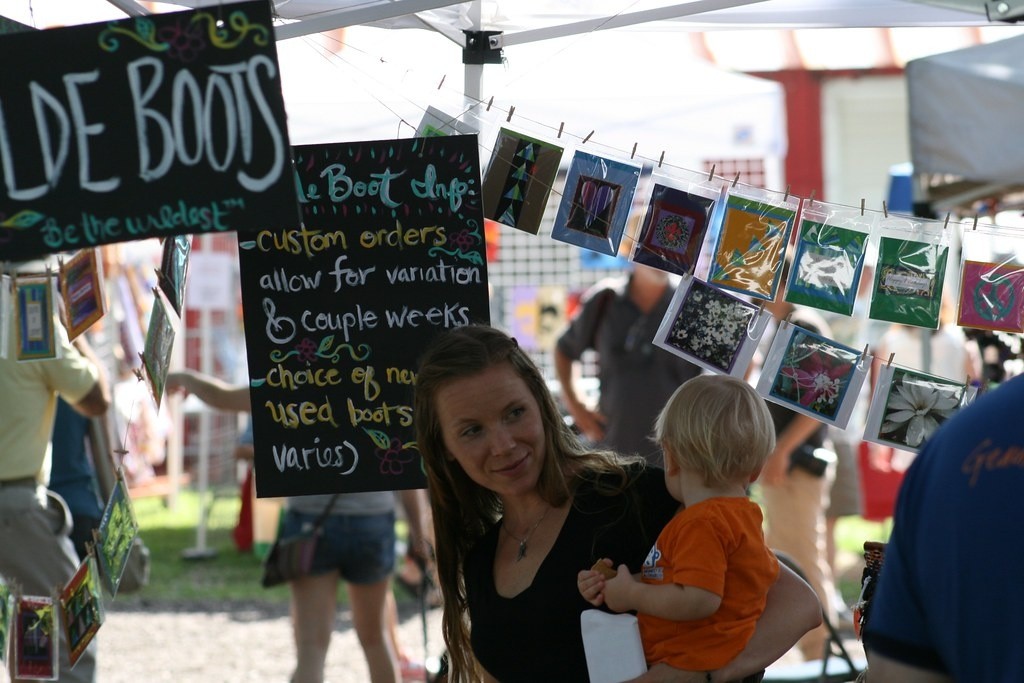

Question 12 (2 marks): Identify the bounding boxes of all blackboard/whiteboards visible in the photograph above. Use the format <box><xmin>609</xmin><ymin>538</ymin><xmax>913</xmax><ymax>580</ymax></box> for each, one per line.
<box><xmin>0</xmin><ymin>0</ymin><xmax>291</xmax><ymax>270</ymax></box>
<box><xmin>237</xmin><ymin>132</ymin><xmax>488</xmax><ymax>504</ymax></box>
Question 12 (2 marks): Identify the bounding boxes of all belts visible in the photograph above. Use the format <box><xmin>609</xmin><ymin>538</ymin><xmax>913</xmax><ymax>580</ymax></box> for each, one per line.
<box><xmin>0</xmin><ymin>477</ymin><xmax>39</xmax><ymax>487</ymax></box>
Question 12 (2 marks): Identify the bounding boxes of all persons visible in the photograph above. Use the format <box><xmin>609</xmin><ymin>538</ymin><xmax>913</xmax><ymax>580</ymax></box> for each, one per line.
<box><xmin>233</xmin><ymin>417</ymin><xmax>255</xmax><ymax>460</ymax></box>
<box><xmin>551</xmin><ymin>254</ymin><xmax>704</xmax><ymax>461</ymax></box>
<box><xmin>578</xmin><ymin>374</ymin><xmax>778</xmax><ymax>668</ymax></box>
<box><xmin>861</xmin><ymin>372</ymin><xmax>1024</xmax><ymax>683</ymax></box>
<box><xmin>753</xmin><ymin>245</ymin><xmax>829</xmax><ymax>659</ymax></box>
<box><xmin>0</xmin><ymin>257</ymin><xmax>111</xmax><ymax>683</ymax></box>
<box><xmin>166</xmin><ymin>370</ymin><xmax>432</xmax><ymax>683</ymax></box>
<box><xmin>417</xmin><ymin>326</ymin><xmax>824</xmax><ymax>683</ymax></box>
<box><xmin>828</xmin><ymin>260</ymin><xmax>1024</xmax><ymax>576</ymax></box>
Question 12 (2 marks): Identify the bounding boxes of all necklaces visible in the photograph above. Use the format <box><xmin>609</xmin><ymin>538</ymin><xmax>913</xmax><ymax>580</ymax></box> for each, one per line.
<box><xmin>502</xmin><ymin>506</ymin><xmax>550</xmax><ymax>560</ymax></box>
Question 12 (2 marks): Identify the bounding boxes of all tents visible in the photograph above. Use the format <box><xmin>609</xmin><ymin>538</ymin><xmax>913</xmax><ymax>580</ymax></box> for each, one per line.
<box><xmin>905</xmin><ymin>33</ymin><xmax>1023</xmax><ymax>377</ymax></box>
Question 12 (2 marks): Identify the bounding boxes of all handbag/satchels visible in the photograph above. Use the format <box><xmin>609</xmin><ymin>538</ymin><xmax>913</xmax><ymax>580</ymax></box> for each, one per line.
<box><xmin>263</xmin><ymin>530</ymin><xmax>313</xmax><ymax>587</ymax></box>
